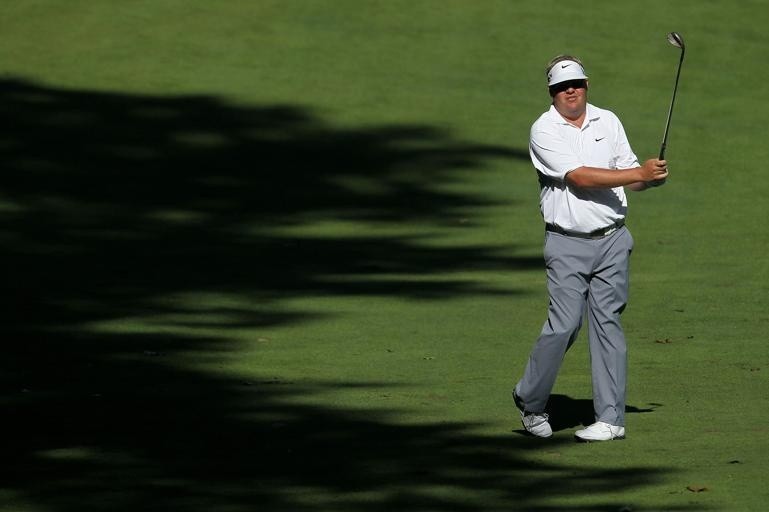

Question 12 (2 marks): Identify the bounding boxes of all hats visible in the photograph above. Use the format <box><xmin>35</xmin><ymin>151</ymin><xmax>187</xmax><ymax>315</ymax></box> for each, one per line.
<box><xmin>547</xmin><ymin>60</ymin><xmax>589</xmax><ymax>86</ymax></box>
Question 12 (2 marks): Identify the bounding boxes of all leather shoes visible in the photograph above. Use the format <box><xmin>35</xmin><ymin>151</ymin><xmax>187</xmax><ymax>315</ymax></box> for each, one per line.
<box><xmin>511</xmin><ymin>386</ymin><xmax>552</xmax><ymax>438</ymax></box>
<box><xmin>574</xmin><ymin>423</ymin><xmax>626</xmax><ymax>441</ymax></box>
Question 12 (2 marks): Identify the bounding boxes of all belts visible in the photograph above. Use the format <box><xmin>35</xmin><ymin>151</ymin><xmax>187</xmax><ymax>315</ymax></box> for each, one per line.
<box><xmin>545</xmin><ymin>221</ymin><xmax>625</xmax><ymax>240</ymax></box>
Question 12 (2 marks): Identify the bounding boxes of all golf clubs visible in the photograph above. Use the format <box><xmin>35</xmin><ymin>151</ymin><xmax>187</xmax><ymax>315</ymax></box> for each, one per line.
<box><xmin>658</xmin><ymin>32</ymin><xmax>686</xmax><ymax>162</ymax></box>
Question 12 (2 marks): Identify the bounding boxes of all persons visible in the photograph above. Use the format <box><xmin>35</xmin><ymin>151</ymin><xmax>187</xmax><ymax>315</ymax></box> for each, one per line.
<box><xmin>511</xmin><ymin>55</ymin><xmax>667</xmax><ymax>442</ymax></box>
